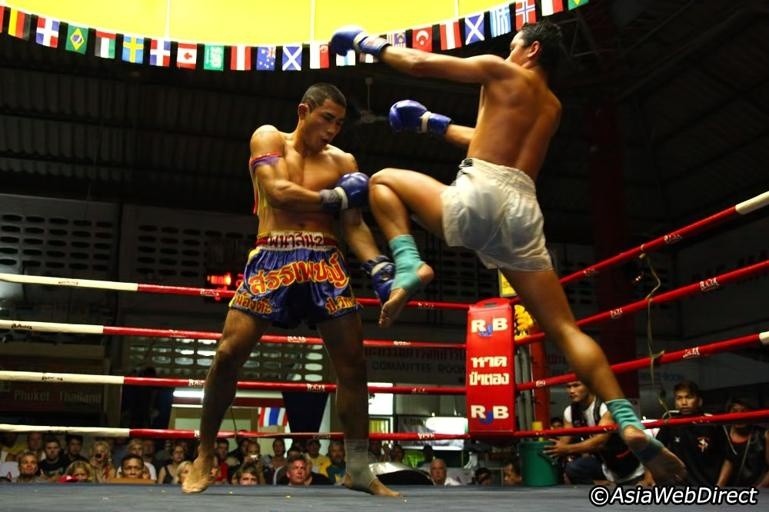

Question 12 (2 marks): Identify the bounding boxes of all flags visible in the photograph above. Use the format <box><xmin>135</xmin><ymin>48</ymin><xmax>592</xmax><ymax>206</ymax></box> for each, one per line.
<box><xmin>0</xmin><ymin>0</ymin><xmax>589</xmax><ymax>72</ymax></box>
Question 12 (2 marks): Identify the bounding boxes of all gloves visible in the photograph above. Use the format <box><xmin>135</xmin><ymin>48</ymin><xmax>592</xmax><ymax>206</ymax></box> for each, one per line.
<box><xmin>329</xmin><ymin>23</ymin><xmax>391</xmax><ymax>65</ymax></box>
<box><xmin>358</xmin><ymin>254</ymin><xmax>398</xmax><ymax>309</ymax></box>
<box><xmin>386</xmin><ymin>98</ymin><xmax>454</xmax><ymax>142</ymax></box>
<box><xmin>322</xmin><ymin>169</ymin><xmax>370</xmax><ymax>214</ymax></box>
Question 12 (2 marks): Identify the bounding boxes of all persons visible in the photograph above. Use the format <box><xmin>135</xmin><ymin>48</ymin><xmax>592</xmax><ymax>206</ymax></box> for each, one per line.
<box><xmin>0</xmin><ymin>365</ymin><xmax>765</xmax><ymax>488</ymax></box>
<box><xmin>329</xmin><ymin>22</ymin><xmax>689</xmax><ymax>487</ymax></box>
<box><xmin>182</xmin><ymin>83</ymin><xmax>402</xmax><ymax>497</ymax></box>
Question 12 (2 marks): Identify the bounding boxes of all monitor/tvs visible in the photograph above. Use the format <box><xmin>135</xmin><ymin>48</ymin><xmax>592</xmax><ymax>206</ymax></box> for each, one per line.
<box><xmin>367</xmin><ymin>380</ymin><xmax>395</xmax><ymax>416</ymax></box>
<box><xmin>424</xmin><ymin>416</ymin><xmax>469</xmax><ymax>452</ymax></box>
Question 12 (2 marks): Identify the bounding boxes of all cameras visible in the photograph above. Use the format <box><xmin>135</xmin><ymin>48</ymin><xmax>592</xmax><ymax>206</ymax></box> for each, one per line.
<box><xmin>96</xmin><ymin>453</ymin><xmax>101</xmax><ymax>458</ymax></box>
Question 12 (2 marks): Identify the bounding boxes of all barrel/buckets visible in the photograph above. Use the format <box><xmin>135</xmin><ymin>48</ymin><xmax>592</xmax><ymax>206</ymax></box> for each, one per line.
<box><xmin>520</xmin><ymin>440</ymin><xmax>561</xmax><ymax>487</ymax></box>
